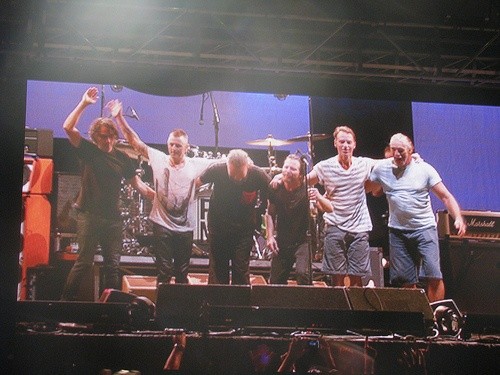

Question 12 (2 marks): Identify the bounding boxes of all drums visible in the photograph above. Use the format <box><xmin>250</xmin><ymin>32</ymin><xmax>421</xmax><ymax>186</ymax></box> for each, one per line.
<box><xmin>125</xmin><ymin>182</ymin><xmax>153</xmax><ymax>235</ymax></box>
<box><xmin>257</xmin><ymin>167</ymin><xmax>282</xmax><ymax>205</ymax></box>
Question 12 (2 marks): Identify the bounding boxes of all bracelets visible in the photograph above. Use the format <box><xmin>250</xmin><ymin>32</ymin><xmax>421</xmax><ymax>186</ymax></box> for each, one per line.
<box><xmin>174</xmin><ymin>343</ymin><xmax>185</xmax><ymax>350</ymax></box>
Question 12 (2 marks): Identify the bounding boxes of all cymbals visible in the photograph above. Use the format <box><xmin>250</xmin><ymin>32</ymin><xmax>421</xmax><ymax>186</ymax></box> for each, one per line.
<box><xmin>284</xmin><ymin>130</ymin><xmax>331</xmax><ymax>145</ymax></box>
<box><xmin>114</xmin><ymin>141</ymin><xmax>148</xmax><ymax>163</ymax></box>
<box><xmin>246</xmin><ymin>134</ymin><xmax>290</xmax><ymax>149</ymax></box>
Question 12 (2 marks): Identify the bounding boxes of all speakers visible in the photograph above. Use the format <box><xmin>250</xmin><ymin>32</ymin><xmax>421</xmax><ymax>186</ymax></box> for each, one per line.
<box><xmin>348</xmin><ymin>286</ymin><xmax>434</xmax><ymax>332</ymax></box>
<box><xmin>436</xmin><ymin>237</ymin><xmax>500</xmax><ymax>324</ymax></box>
<box><xmin>249</xmin><ymin>284</ymin><xmax>351</xmax><ymax>324</ymax></box>
<box><xmin>153</xmin><ymin>283</ymin><xmax>250</xmax><ymax>328</ymax></box>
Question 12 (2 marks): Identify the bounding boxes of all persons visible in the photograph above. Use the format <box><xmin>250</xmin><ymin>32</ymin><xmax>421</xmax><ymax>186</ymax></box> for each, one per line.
<box><xmin>164</xmin><ymin>335</ymin><xmax>186</xmax><ymax>375</ymax></box>
<box><xmin>106</xmin><ymin>99</ymin><xmax>228</xmax><ymax>283</ymax></box>
<box><xmin>270</xmin><ymin>126</ymin><xmax>422</xmax><ymax>288</ymax></box>
<box><xmin>264</xmin><ymin>154</ymin><xmax>334</xmax><ymax>285</ymax></box>
<box><xmin>61</xmin><ymin>87</ymin><xmax>156</xmax><ymax>301</ymax></box>
<box><xmin>276</xmin><ymin>336</ymin><xmax>500</xmax><ymax>375</ymax></box>
<box><xmin>366</xmin><ymin>132</ymin><xmax>466</xmax><ymax>303</ymax></box>
<box><xmin>196</xmin><ymin>149</ymin><xmax>273</xmax><ymax>285</ymax></box>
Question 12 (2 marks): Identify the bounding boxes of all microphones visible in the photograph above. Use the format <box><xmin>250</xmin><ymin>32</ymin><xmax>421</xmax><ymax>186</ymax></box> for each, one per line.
<box><xmin>129</xmin><ymin>106</ymin><xmax>139</xmax><ymax>120</ymax></box>
<box><xmin>301</xmin><ymin>156</ymin><xmax>310</xmax><ymax>165</ymax></box>
<box><xmin>200</xmin><ymin>103</ymin><xmax>204</xmax><ymax>125</ymax></box>
